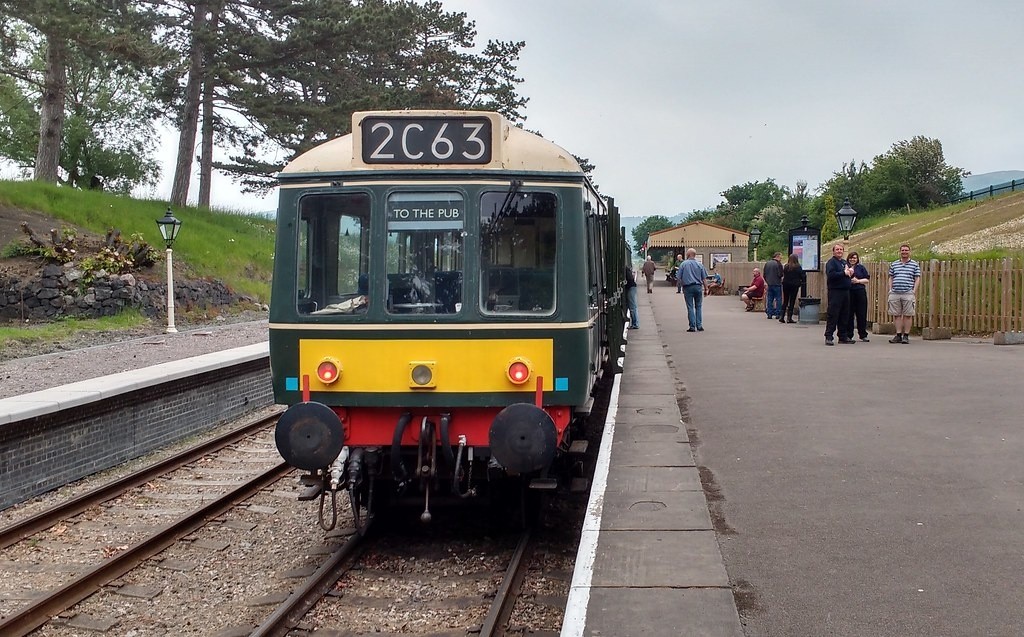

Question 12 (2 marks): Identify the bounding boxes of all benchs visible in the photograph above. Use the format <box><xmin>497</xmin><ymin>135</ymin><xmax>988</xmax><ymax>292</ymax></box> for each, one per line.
<box><xmin>354</xmin><ymin>264</ymin><xmax>557</xmax><ymax>313</ymax></box>
<box><xmin>714</xmin><ymin>278</ymin><xmax>726</xmax><ymax>294</ymax></box>
<box><xmin>751</xmin><ymin>285</ymin><xmax>766</xmax><ymax>311</ymax></box>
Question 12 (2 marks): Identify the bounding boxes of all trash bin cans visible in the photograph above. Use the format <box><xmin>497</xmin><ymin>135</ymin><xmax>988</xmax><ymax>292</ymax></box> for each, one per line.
<box><xmin>739</xmin><ymin>286</ymin><xmax>750</xmax><ymax>301</ymax></box>
<box><xmin>798</xmin><ymin>298</ymin><xmax>821</xmax><ymax>324</ymax></box>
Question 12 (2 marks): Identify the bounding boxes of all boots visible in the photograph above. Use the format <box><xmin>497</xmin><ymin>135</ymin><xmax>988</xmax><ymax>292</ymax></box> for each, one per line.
<box><xmin>786</xmin><ymin>311</ymin><xmax>796</xmax><ymax>323</ymax></box>
<box><xmin>779</xmin><ymin>310</ymin><xmax>785</xmax><ymax>323</ymax></box>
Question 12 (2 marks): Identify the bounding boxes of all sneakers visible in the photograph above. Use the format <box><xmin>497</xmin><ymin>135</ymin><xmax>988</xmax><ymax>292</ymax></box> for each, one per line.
<box><xmin>889</xmin><ymin>337</ymin><xmax>902</xmax><ymax>343</ymax></box>
<box><xmin>902</xmin><ymin>337</ymin><xmax>909</xmax><ymax>344</ymax></box>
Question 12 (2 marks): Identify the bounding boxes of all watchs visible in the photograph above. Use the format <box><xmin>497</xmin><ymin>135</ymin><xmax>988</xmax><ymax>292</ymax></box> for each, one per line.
<box><xmin>704</xmin><ymin>288</ymin><xmax>708</xmax><ymax>290</ymax></box>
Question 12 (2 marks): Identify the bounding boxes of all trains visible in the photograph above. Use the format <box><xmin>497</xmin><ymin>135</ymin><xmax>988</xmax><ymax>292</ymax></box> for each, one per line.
<box><xmin>268</xmin><ymin>107</ymin><xmax>633</xmax><ymax>539</ymax></box>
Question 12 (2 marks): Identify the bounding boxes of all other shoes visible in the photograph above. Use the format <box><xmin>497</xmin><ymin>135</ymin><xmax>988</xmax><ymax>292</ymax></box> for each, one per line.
<box><xmin>863</xmin><ymin>337</ymin><xmax>869</xmax><ymax>342</ymax></box>
<box><xmin>687</xmin><ymin>328</ymin><xmax>695</xmax><ymax>332</ymax></box>
<box><xmin>838</xmin><ymin>337</ymin><xmax>855</xmax><ymax>344</ymax></box>
<box><xmin>768</xmin><ymin>314</ymin><xmax>772</xmax><ymax>319</ymax></box>
<box><xmin>746</xmin><ymin>304</ymin><xmax>754</xmax><ymax>311</ymax></box>
<box><xmin>627</xmin><ymin>326</ymin><xmax>639</xmax><ymax>329</ymax></box>
<box><xmin>825</xmin><ymin>337</ymin><xmax>834</xmax><ymax>345</ymax></box>
<box><xmin>776</xmin><ymin>315</ymin><xmax>779</xmax><ymax>319</ymax></box>
<box><xmin>697</xmin><ymin>326</ymin><xmax>704</xmax><ymax>331</ymax></box>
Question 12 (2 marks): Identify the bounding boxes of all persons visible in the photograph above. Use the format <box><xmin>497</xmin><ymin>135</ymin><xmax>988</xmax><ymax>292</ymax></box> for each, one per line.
<box><xmin>674</xmin><ymin>254</ymin><xmax>684</xmax><ymax>294</ymax></box>
<box><xmin>741</xmin><ymin>267</ymin><xmax>764</xmax><ymax>312</ymax></box>
<box><xmin>708</xmin><ymin>270</ymin><xmax>723</xmax><ymax>295</ymax></box>
<box><xmin>824</xmin><ymin>242</ymin><xmax>856</xmax><ymax>346</ymax></box>
<box><xmin>624</xmin><ymin>266</ymin><xmax>640</xmax><ymax>330</ymax></box>
<box><xmin>779</xmin><ymin>253</ymin><xmax>806</xmax><ymax>323</ymax></box>
<box><xmin>642</xmin><ymin>255</ymin><xmax>657</xmax><ymax>294</ymax></box>
<box><xmin>887</xmin><ymin>245</ymin><xmax>921</xmax><ymax>344</ymax></box>
<box><xmin>847</xmin><ymin>252</ymin><xmax>871</xmax><ymax>343</ymax></box>
<box><xmin>762</xmin><ymin>252</ymin><xmax>784</xmax><ymax>320</ymax></box>
<box><xmin>676</xmin><ymin>248</ymin><xmax>709</xmax><ymax>333</ymax></box>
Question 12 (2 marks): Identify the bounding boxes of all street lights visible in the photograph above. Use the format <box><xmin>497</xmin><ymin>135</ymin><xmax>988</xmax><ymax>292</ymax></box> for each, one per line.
<box><xmin>157</xmin><ymin>206</ymin><xmax>183</xmax><ymax>334</ymax></box>
<box><xmin>836</xmin><ymin>199</ymin><xmax>858</xmax><ymax>261</ymax></box>
<box><xmin>749</xmin><ymin>224</ymin><xmax>762</xmax><ymax>262</ymax></box>
